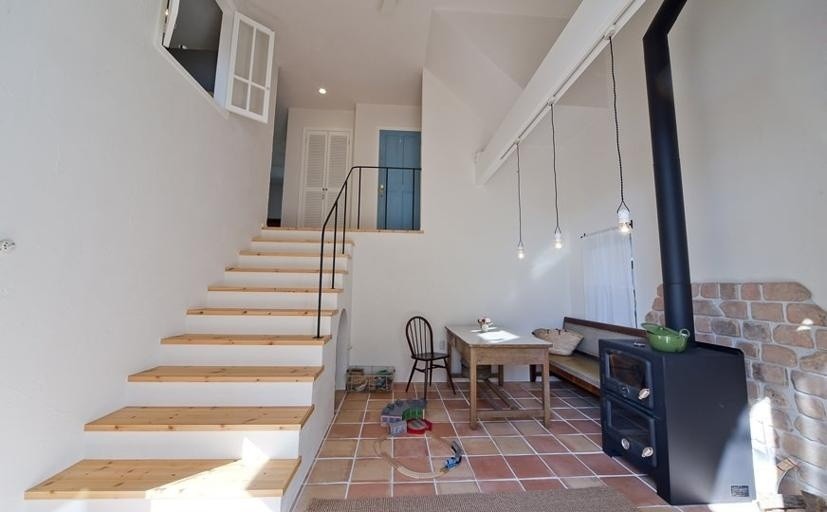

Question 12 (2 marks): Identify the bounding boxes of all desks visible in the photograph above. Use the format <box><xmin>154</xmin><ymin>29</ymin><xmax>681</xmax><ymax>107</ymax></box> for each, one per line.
<box><xmin>445</xmin><ymin>324</ymin><xmax>553</xmax><ymax>431</ymax></box>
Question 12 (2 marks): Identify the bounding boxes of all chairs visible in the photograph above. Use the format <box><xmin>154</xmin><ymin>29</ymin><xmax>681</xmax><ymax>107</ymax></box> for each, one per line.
<box><xmin>406</xmin><ymin>316</ymin><xmax>456</xmax><ymax>401</ymax></box>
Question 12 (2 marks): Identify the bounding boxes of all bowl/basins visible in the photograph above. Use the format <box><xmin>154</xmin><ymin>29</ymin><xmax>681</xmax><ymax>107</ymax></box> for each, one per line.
<box><xmin>648</xmin><ymin>332</ymin><xmax>691</xmax><ymax>352</ymax></box>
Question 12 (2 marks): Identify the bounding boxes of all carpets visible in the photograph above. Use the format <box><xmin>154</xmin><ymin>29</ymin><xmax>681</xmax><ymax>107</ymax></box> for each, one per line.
<box><xmin>305</xmin><ymin>486</ymin><xmax>637</xmax><ymax>511</ymax></box>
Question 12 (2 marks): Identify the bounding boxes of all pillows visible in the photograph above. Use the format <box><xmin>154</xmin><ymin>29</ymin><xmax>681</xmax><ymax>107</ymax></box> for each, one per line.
<box><xmin>532</xmin><ymin>329</ymin><xmax>585</xmax><ymax>357</ymax></box>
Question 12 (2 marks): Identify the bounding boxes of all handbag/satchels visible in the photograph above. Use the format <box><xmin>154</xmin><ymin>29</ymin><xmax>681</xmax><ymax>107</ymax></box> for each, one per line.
<box><xmin>532</xmin><ymin>328</ymin><xmax>584</xmax><ymax>355</ymax></box>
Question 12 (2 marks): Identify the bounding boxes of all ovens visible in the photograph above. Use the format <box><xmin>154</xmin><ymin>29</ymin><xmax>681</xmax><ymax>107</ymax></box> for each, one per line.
<box><xmin>599</xmin><ymin>340</ymin><xmax>753</xmax><ymax>505</ymax></box>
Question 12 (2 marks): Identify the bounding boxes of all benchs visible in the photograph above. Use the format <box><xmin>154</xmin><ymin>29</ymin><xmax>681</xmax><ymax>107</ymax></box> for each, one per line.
<box><xmin>529</xmin><ymin>317</ymin><xmax>647</xmax><ymax>398</ymax></box>
